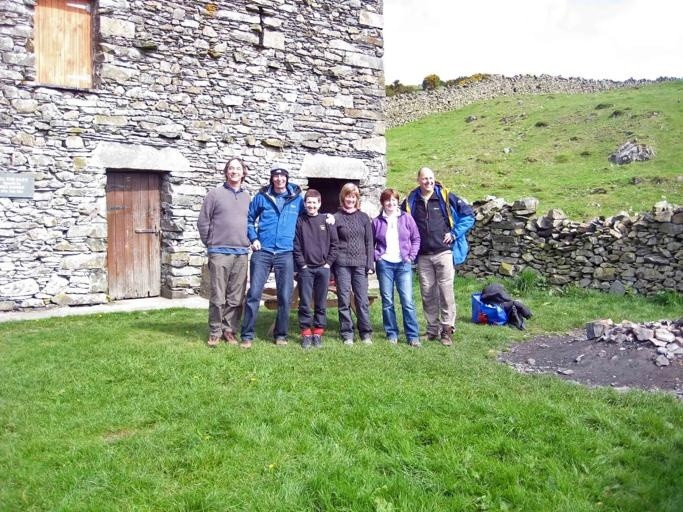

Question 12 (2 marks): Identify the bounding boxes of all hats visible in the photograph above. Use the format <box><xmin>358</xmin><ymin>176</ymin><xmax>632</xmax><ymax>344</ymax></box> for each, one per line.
<box><xmin>271</xmin><ymin>164</ymin><xmax>289</xmax><ymax>176</ymax></box>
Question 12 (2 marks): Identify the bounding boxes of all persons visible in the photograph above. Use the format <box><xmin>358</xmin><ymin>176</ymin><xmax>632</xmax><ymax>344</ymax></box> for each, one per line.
<box><xmin>399</xmin><ymin>167</ymin><xmax>476</xmax><ymax>346</ymax></box>
<box><xmin>197</xmin><ymin>158</ymin><xmax>251</xmax><ymax>347</ymax></box>
<box><xmin>294</xmin><ymin>189</ymin><xmax>340</xmax><ymax>348</ymax></box>
<box><xmin>331</xmin><ymin>183</ymin><xmax>376</xmax><ymax>345</ymax></box>
<box><xmin>371</xmin><ymin>189</ymin><xmax>422</xmax><ymax>349</ymax></box>
<box><xmin>239</xmin><ymin>163</ymin><xmax>335</xmax><ymax>348</ymax></box>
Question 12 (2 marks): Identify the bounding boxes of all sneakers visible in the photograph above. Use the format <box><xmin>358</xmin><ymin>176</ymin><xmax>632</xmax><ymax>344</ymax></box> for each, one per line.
<box><xmin>208</xmin><ymin>335</ymin><xmax>219</xmax><ymax>347</ymax></box>
<box><xmin>362</xmin><ymin>338</ymin><xmax>372</xmax><ymax>343</ymax></box>
<box><xmin>240</xmin><ymin>337</ymin><xmax>252</xmax><ymax>348</ymax></box>
<box><xmin>387</xmin><ymin>339</ymin><xmax>397</xmax><ymax>344</ymax></box>
<box><xmin>302</xmin><ymin>335</ymin><xmax>313</xmax><ymax>348</ymax></box>
<box><xmin>419</xmin><ymin>334</ymin><xmax>434</xmax><ymax>341</ymax></box>
<box><xmin>410</xmin><ymin>342</ymin><xmax>422</xmax><ymax>348</ymax></box>
<box><xmin>440</xmin><ymin>335</ymin><xmax>453</xmax><ymax>346</ymax></box>
<box><xmin>225</xmin><ymin>333</ymin><xmax>238</xmax><ymax>345</ymax></box>
<box><xmin>313</xmin><ymin>334</ymin><xmax>323</xmax><ymax>349</ymax></box>
<box><xmin>276</xmin><ymin>336</ymin><xmax>287</xmax><ymax>345</ymax></box>
<box><xmin>343</xmin><ymin>338</ymin><xmax>354</xmax><ymax>345</ymax></box>
